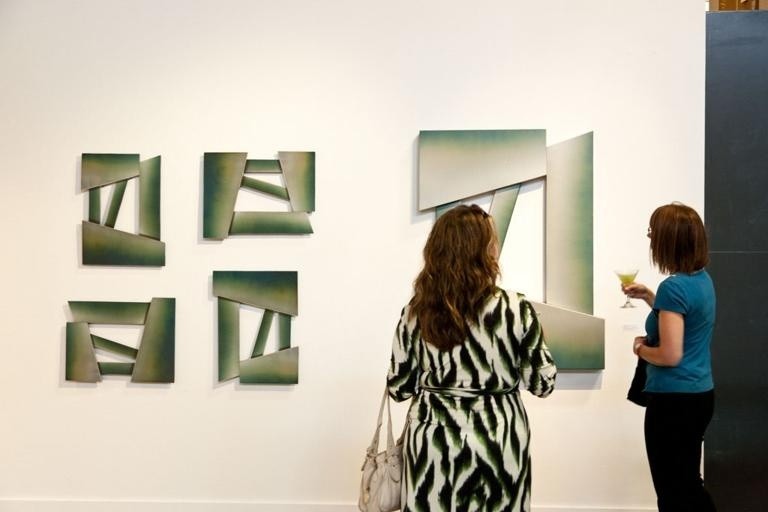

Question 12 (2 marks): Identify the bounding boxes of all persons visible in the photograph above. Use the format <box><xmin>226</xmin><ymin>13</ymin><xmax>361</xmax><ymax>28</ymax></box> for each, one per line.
<box><xmin>384</xmin><ymin>202</ymin><xmax>559</xmax><ymax>511</ymax></box>
<box><xmin>619</xmin><ymin>202</ymin><xmax>719</xmax><ymax>511</ymax></box>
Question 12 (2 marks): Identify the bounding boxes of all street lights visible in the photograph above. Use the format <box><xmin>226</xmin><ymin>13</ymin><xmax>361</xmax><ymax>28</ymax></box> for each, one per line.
<box><xmin>354</xmin><ymin>385</ymin><xmax>402</xmax><ymax>512</ymax></box>
<box><xmin>626</xmin><ymin>356</ymin><xmax>648</xmax><ymax>407</ymax></box>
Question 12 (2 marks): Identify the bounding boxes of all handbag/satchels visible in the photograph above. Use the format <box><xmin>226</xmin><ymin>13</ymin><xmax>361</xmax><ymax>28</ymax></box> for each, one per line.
<box><xmin>635</xmin><ymin>342</ymin><xmax>642</xmax><ymax>355</ymax></box>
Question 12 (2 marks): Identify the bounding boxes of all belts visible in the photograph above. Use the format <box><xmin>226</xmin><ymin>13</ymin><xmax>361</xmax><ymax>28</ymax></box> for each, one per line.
<box><xmin>615</xmin><ymin>268</ymin><xmax>639</xmax><ymax>309</ymax></box>
